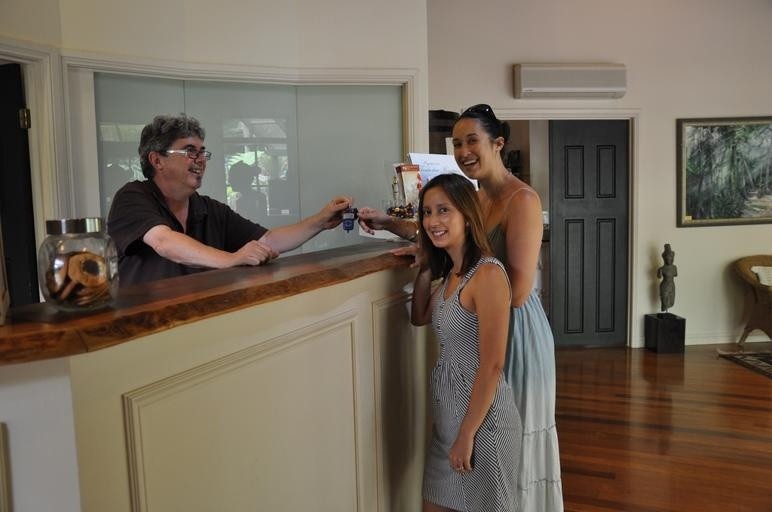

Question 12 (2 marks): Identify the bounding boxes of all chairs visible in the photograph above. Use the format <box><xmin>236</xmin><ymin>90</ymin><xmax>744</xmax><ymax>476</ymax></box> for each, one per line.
<box><xmin>733</xmin><ymin>255</ymin><xmax>772</xmax><ymax>352</ymax></box>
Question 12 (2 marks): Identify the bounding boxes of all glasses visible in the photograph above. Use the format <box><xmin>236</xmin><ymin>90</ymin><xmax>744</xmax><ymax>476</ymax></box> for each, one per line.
<box><xmin>165</xmin><ymin>147</ymin><xmax>212</xmax><ymax>159</ymax></box>
<box><xmin>468</xmin><ymin>105</ymin><xmax>496</xmax><ymax>119</ymax></box>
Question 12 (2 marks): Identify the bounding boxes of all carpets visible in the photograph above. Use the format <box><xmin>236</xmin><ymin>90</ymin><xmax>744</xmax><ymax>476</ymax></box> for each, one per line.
<box><xmin>718</xmin><ymin>350</ymin><xmax>771</xmax><ymax>380</ymax></box>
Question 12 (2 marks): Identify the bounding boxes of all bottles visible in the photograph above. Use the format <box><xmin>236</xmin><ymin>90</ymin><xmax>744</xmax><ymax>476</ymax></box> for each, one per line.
<box><xmin>392</xmin><ymin>176</ymin><xmax>399</xmax><ymax>201</ymax></box>
<box><xmin>38</xmin><ymin>217</ymin><xmax>120</xmax><ymax>314</ymax></box>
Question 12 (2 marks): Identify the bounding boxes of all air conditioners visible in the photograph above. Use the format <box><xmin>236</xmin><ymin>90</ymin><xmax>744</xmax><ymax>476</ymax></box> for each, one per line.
<box><xmin>512</xmin><ymin>62</ymin><xmax>627</xmax><ymax>99</ymax></box>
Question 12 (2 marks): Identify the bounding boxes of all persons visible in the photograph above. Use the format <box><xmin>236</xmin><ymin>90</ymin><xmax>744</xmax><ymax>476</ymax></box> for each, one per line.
<box><xmin>656</xmin><ymin>242</ymin><xmax>678</xmax><ymax>311</ymax></box>
<box><xmin>388</xmin><ymin>173</ymin><xmax>523</xmax><ymax>511</ymax></box>
<box><xmin>228</xmin><ymin>160</ymin><xmax>267</xmax><ymax>218</ymax></box>
<box><xmin>108</xmin><ymin>112</ymin><xmax>354</xmax><ymax>289</ymax></box>
<box><xmin>357</xmin><ymin>105</ymin><xmax>563</xmax><ymax>512</ymax></box>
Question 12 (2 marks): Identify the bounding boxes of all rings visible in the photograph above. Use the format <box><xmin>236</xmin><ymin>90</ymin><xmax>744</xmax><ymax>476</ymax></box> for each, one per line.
<box><xmin>460</xmin><ymin>467</ymin><xmax>464</xmax><ymax>469</ymax></box>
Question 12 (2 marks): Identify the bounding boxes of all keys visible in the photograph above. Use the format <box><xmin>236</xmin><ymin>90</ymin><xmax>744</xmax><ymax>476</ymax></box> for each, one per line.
<box><xmin>342</xmin><ymin>206</ymin><xmax>359</xmax><ymax>233</ymax></box>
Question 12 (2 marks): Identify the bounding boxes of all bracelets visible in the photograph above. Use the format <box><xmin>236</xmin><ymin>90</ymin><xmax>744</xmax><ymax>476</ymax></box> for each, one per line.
<box><xmin>409</xmin><ymin>220</ymin><xmax>419</xmax><ymax>242</ymax></box>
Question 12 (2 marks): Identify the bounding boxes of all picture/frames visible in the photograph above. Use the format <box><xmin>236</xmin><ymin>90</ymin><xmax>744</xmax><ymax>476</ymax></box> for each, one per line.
<box><xmin>676</xmin><ymin>117</ymin><xmax>771</xmax><ymax>228</ymax></box>
